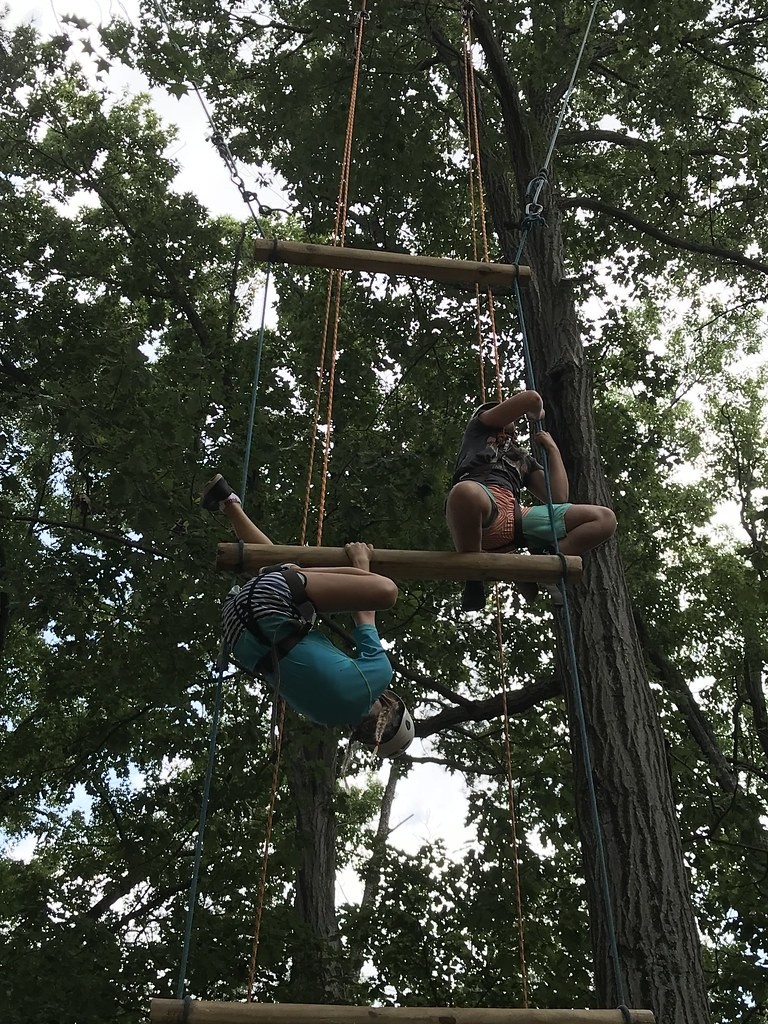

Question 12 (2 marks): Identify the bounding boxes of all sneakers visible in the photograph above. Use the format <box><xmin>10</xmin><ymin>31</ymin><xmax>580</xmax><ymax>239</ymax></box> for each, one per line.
<box><xmin>200</xmin><ymin>474</ymin><xmax>236</xmax><ymax>511</ymax></box>
<box><xmin>260</xmin><ymin>561</ymin><xmax>296</xmax><ymax>574</ymax></box>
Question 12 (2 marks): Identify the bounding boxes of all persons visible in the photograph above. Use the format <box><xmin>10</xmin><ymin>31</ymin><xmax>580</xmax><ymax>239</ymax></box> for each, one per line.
<box><xmin>446</xmin><ymin>391</ymin><xmax>617</xmax><ymax>611</ymax></box>
<box><xmin>200</xmin><ymin>474</ymin><xmax>414</xmax><ymax>759</ymax></box>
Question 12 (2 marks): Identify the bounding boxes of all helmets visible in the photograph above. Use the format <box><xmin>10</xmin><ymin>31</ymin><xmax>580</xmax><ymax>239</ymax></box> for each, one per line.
<box><xmin>356</xmin><ymin>690</ymin><xmax>415</xmax><ymax>758</ymax></box>
<box><xmin>470</xmin><ymin>401</ymin><xmax>500</xmax><ymax>418</ymax></box>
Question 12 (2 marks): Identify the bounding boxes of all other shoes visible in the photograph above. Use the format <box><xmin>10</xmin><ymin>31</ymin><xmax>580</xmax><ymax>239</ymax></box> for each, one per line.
<box><xmin>516</xmin><ymin>581</ymin><xmax>539</xmax><ymax>601</ymax></box>
<box><xmin>461</xmin><ymin>581</ymin><xmax>486</xmax><ymax>610</ymax></box>
